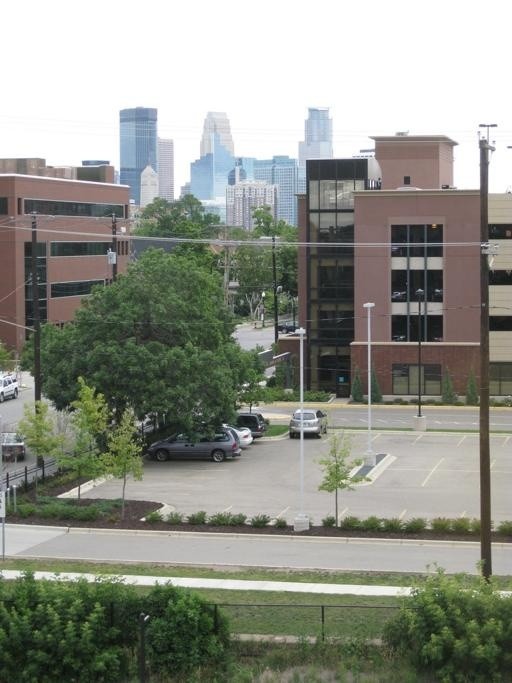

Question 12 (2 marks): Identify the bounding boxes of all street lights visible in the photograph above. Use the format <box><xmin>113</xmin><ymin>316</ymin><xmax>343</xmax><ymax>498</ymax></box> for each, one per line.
<box><xmin>294</xmin><ymin>327</ymin><xmax>310</xmax><ymax>531</ymax></box>
<box><xmin>278</xmin><ymin>323</ymin><xmax>297</xmax><ymax>333</ymax></box>
<box><xmin>415</xmin><ymin>288</ymin><xmax>425</xmax><ymax>417</ymax></box>
<box><xmin>274</xmin><ymin>285</ymin><xmax>283</xmax><ymax>341</ymax></box>
<box><xmin>363</xmin><ymin>301</ymin><xmax>376</xmax><ymax>466</ymax></box>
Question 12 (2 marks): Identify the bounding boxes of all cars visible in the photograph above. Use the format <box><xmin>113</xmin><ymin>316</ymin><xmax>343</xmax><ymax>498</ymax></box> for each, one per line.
<box><xmin>0</xmin><ymin>431</ymin><xmax>25</xmax><ymax>462</ymax></box>
<box><xmin>289</xmin><ymin>408</ymin><xmax>328</xmax><ymax>439</ymax></box>
<box><xmin>0</xmin><ymin>374</ymin><xmax>19</xmax><ymax>402</ymax></box>
<box><xmin>146</xmin><ymin>413</ymin><xmax>266</xmax><ymax>462</ymax></box>
